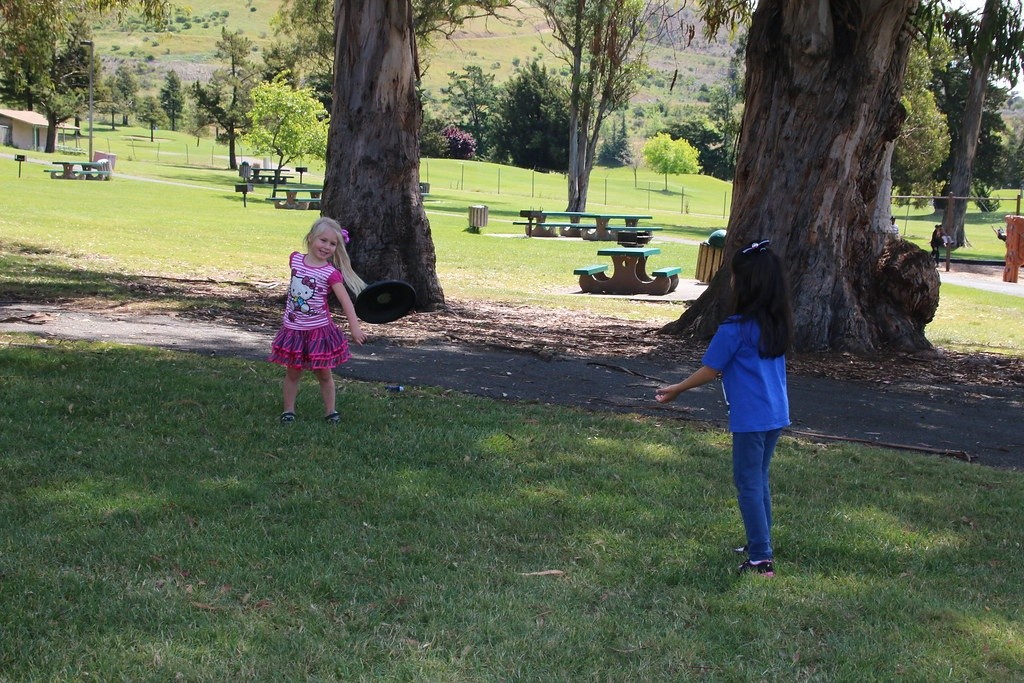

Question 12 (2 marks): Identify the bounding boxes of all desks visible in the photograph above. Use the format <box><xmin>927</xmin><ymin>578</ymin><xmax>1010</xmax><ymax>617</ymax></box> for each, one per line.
<box><xmin>578</xmin><ymin>247</ymin><xmax>678</xmax><ymax>296</ymax></box>
<box><xmin>525</xmin><ymin>211</ymin><xmax>652</xmax><ymax>241</ymax></box>
<box><xmin>274</xmin><ymin>188</ymin><xmax>323</xmax><ymax>210</ymax></box>
<box><xmin>250</xmin><ymin>167</ymin><xmax>290</xmax><ymax>183</ymax></box>
<box><xmin>51</xmin><ymin>161</ymin><xmax>109</xmax><ymax>181</ymax></box>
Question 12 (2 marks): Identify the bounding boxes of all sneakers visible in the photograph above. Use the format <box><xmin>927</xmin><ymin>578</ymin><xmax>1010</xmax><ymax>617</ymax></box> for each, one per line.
<box><xmin>734</xmin><ymin>545</ymin><xmax>748</xmax><ymax>554</ymax></box>
<box><xmin>738</xmin><ymin>558</ymin><xmax>773</xmax><ymax>577</ymax></box>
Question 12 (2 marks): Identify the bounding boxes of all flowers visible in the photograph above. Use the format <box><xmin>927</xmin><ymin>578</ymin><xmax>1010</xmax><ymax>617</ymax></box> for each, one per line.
<box><xmin>340</xmin><ymin>228</ymin><xmax>351</xmax><ymax>243</ymax></box>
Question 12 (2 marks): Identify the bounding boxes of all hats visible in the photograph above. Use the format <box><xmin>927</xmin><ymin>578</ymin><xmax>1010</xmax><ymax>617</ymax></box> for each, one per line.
<box><xmin>935</xmin><ymin>225</ymin><xmax>941</xmax><ymax>229</ymax></box>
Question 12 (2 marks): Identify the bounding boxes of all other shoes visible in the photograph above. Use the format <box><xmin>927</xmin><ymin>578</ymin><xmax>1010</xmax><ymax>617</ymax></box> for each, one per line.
<box><xmin>280</xmin><ymin>412</ymin><xmax>296</xmax><ymax>422</ymax></box>
<box><xmin>325</xmin><ymin>413</ymin><xmax>341</xmax><ymax>424</ymax></box>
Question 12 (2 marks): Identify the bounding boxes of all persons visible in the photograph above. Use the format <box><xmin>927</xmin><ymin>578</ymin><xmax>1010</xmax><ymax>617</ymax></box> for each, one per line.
<box><xmin>931</xmin><ymin>225</ymin><xmax>941</xmax><ymax>268</ymax></box>
<box><xmin>269</xmin><ymin>216</ymin><xmax>368</xmax><ymax>424</ymax></box>
<box><xmin>655</xmin><ymin>240</ymin><xmax>793</xmax><ymax>576</ymax></box>
<box><xmin>991</xmin><ymin>225</ymin><xmax>1006</xmax><ymax>241</ymax></box>
<box><xmin>891</xmin><ymin>216</ymin><xmax>899</xmax><ymax>237</ymax></box>
<box><xmin>938</xmin><ymin>225</ymin><xmax>955</xmax><ymax>246</ymax></box>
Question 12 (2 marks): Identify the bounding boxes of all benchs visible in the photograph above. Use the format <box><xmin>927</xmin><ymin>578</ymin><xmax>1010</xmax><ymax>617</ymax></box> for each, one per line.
<box><xmin>573</xmin><ymin>264</ymin><xmax>609</xmax><ymax>276</ymax></box>
<box><xmin>251</xmin><ymin>174</ymin><xmax>274</xmax><ymax>178</ymax></box>
<box><xmin>266</xmin><ymin>197</ymin><xmax>287</xmax><ymax>201</ymax></box>
<box><xmin>652</xmin><ymin>267</ymin><xmax>682</xmax><ymax>277</ymax></box>
<box><xmin>293</xmin><ymin>197</ymin><xmax>322</xmax><ymax>202</ymax></box>
<box><xmin>571</xmin><ymin>224</ymin><xmax>664</xmax><ymax>231</ymax></box>
<box><xmin>280</xmin><ymin>175</ymin><xmax>294</xmax><ymax>177</ymax></box>
<box><xmin>79</xmin><ymin>170</ymin><xmax>111</xmax><ymax>174</ymax></box>
<box><xmin>43</xmin><ymin>169</ymin><xmax>78</xmax><ymax>173</ymax></box>
<box><xmin>513</xmin><ymin>221</ymin><xmax>576</xmax><ymax>228</ymax></box>
